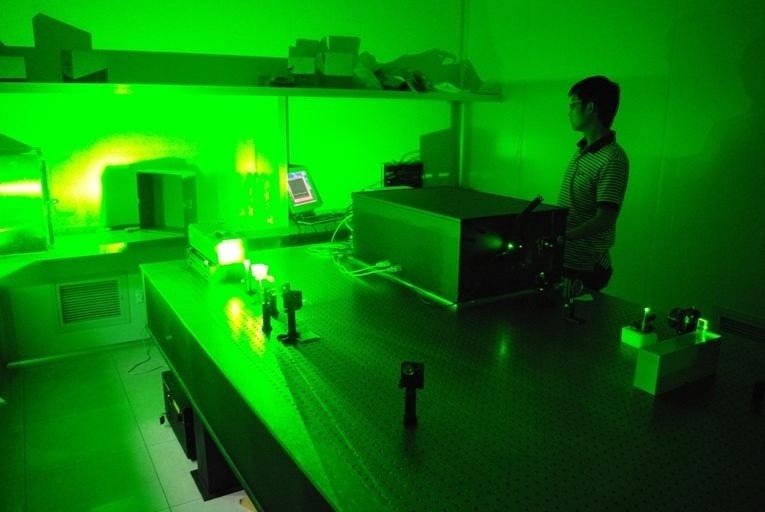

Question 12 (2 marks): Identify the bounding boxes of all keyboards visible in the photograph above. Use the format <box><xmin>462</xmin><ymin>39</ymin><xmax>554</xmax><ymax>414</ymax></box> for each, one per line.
<box><xmin>298</xmin><ymin>213</ymin><xmax>347</xmax><ymax>225</ymax></box>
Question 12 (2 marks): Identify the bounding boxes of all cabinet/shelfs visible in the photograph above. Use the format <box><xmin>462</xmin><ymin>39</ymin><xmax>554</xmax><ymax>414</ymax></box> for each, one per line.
<box><xmin>1</xmin><ymin>13</ymin><xmax>503</xmax><ymax>361</ymax></box>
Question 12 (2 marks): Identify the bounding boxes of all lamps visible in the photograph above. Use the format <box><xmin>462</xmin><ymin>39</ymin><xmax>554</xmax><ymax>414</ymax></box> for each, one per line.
<box><xmin>214</xmin><ymin>239</ymin><xmax>276</xmax><ymax>291</ymax></box>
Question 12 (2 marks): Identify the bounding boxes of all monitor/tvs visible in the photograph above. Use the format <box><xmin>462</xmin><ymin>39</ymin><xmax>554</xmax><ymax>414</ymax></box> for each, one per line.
<box><xmin>288</xmin><ymin>167</ymin><xmax>322</xmax><ymax>217</ymax></box>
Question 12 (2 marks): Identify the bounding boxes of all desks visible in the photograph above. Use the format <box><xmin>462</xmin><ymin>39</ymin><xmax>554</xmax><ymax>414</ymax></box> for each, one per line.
<box><xmin>138</xmin><ymin>242</ymin><xmax>765</xmax><ymax>512</ymax></box>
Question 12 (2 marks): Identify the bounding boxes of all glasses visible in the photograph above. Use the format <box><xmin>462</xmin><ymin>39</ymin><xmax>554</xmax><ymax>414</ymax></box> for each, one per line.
<box><xmin>569</xmin><ymin>103</ymin><xmax>581</xmax><ymax>110</ymax></box>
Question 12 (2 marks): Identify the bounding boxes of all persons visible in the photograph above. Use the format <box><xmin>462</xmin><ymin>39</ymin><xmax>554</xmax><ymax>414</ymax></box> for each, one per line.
<box><xmin>556</xmin><ymin>75</ymin><xmax>629</xmax><ymax>290</ymax></box>
